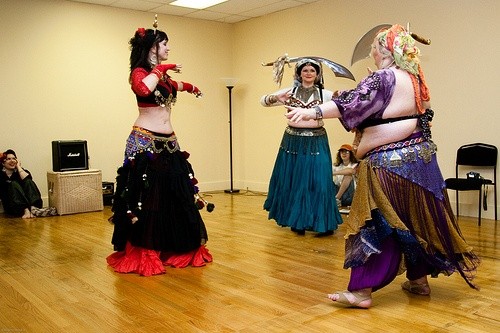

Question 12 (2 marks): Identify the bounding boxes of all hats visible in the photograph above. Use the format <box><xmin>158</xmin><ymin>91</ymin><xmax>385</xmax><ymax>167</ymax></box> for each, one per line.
<box><xmin>295</xmin><ymin>59</ymin><xmax>323</xmax><ymax>84</ymax></box>
<box><xmin>338</xmin><ymin>144</ymin><xmax>352</xmax><ymax>151</ymax></box>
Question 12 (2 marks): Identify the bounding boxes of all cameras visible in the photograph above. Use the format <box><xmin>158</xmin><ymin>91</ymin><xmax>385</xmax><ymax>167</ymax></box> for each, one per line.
<box><xmin>466</xmin><ymin>172</ymin><xmax>480</xmax><ymax>179</ymax></box>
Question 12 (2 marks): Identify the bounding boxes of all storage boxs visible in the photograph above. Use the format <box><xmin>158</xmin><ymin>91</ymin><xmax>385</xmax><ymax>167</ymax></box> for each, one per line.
<box><xmin>47</xmin><ymin>171</ymin><xmax>104</xmax><ymax>216</ymax></box>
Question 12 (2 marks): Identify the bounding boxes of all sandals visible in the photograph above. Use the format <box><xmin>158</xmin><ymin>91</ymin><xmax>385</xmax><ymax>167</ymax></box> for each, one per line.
<box><xmin>401</xmin><ymin>281</ymin><xmax>431</xmax><ymax>295</ymax></box>
<box><xmin>326</xmin><ymin>290</ymin><xmax>371</xmax><ymax>307</ymax></box>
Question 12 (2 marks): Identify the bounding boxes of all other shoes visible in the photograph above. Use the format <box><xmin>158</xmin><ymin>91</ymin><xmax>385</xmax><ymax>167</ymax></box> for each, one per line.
<box><xmin>291</xmin><ymin>227</ymin><xmax>305</xmax><ymax>235</ymax></box>
<box><xmin>316</xmin><ymin>230</ymin><xmax>333</xmax><ymax>237</ymax></box>
<box><xmin>336</xmin><ymin>199</ymin><xmax>342</xmax><ymax>210</ymax></box>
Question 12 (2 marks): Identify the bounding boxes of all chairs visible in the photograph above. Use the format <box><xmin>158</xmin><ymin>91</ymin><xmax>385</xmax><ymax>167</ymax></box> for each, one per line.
<box><xmin>445</xmin><ymin>143</ymin><xmax>498</xmax><ymax>226</ymax></box>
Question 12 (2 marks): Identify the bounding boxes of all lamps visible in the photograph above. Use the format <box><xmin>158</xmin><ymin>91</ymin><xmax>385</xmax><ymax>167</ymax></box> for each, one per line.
<box><xmin>222</xmin><ymin>79</ymin><xmax>241</xmax><ymax>193</ymax></box>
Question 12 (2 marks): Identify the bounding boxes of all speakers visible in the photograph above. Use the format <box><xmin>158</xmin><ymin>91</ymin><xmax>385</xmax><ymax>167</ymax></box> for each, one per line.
<box><xmin>53</xmin><ymin>140</ymin><xmax>89</xmax><ymax>172</ymax></box>
<box><xmin>102</xmin><ymin>182</ymin><xmax>114</xmax><ymax>206</ymax></box>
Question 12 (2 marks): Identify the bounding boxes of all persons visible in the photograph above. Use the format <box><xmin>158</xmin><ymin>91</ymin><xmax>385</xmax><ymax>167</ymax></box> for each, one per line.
<box><xmin>0</xmin><ymin>149</ymin><xmax>43</xmax><ymax>218</ymax></box>
<box><xmin>332</xmin><ymin>144</ymin><xmax>358</xmax><ymax>206</ymax></box>
<box><xmin>284</xmin><ymin>24</ymin><xmax>481</xmax><ymax>307</ymax></box>
<box><xmin>106</xmin><ymin>14</ymin><xmax>215</xmax><ymax>276</ymax></box>
<box><xmin>260</xmin><ymin>57</ymin><xmax>348</xmax><ymax>233</ymax></box>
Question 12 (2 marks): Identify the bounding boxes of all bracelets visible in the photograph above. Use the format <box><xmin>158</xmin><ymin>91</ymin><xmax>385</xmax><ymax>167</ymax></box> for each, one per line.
<box><xmin>313</xmin><ymin>105</ymin><xmax>323</xmax><ymax>121</ymax></box>
<box><xmin>269</xmin><ymin>95</ymin><xmax>277</xmax><ymax>104</ymax></box>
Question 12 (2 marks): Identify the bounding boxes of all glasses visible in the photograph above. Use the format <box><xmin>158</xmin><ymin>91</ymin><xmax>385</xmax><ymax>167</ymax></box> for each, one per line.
<box><xmin>340</xmin><ymin>150</ymin><xmax>350</xmax><ymax>154</ymax></box>
